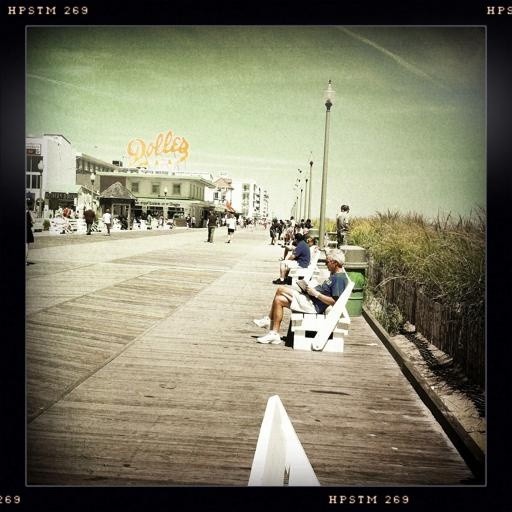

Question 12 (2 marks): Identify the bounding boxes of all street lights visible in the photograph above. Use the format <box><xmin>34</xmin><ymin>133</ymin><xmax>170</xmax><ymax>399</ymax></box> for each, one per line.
<box><xmin>319</xmin><ymin>79</ymin><xmax>335</xmax><ymax>249</ymax></box>
<box><xmin>307</xmin><ymin>150</ymin><xmax>315</xmax><ymax>219</ymax></box>
<box><xmin>163</xmin><ymin>186</ymin><xmax>167</xmax><ymax>220</ymax></box>
<box><xmin>291</xmin><ymin>188</ymin><xmax>303</xmax><ymax>220</ymax></box>
<box><xmin>38</xmin><ymin>160</ymin><xmax>45</xmax><ymax>217</ymax></box>
<box><xmin>90</xmin><ymin>172</ymin><xmax>95</xmax><ymax>209</ymax></box>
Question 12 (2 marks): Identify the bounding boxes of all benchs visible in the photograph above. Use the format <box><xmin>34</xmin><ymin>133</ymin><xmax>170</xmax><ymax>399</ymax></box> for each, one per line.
<box><xmin>288</xmin><ymin>245</ymin><xmax>355</xmax><ymax>353</ymax></box>
<box><xmin>247</xmin><ymin>395</ymin><xmax>320</xmax><ymax>488</ymax></box>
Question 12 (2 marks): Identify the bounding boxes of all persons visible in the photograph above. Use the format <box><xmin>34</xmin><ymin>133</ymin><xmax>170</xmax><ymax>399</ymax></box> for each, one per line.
<box><xmin>57</xmin><ymin>205</ymin><xmax>72</xmax><ymax>218</ymax></box>
<box><xmin>83</xmin><ymin>206</ymin><xmax>97</xmax><ymax>235</ymax></box>
<box><xmin>206</xmin><ymin>210</ymin><xmax>218</xmax><ymax>244</ymax></box>
<box><xmin>338</xmin><ymin>205</ymin><xmax>351</xmax><ymax>249</ymax></box>
<box><xmin>103</xmin><ymin>208</ymin><xmax>112</xmax><ymax>237</ymax></box>
<box><xmin>186</xmin><ymin>214</ymin><xmax>191</xmax><ymax>230</ymax></box>
<box><xmin>238</xmin><ymin>216</ymin><xmax>317</xmax><ymax>284</ymax></box>
<box><xmin>192</xmin><ymin>216</ymin><xmax>196</xmax><ymax>228</ymax></box>
<box><xmin>335</xmin><ymin>205</ymin><xmax>345</xmax><ymax>217</ymax></box>
<box><xmin>25</xmin><ymin>209</ymin><xmax>35</xmax><ymax>268</ymax></box>
<box><xmin>253</xmin><ymin>249</ymin><xmax>348</xmax><ymax>344</ymax></box>
<box><xmin>225</xmin><ymin>213</ymin><xmax>236</xmax><ymax>244</ymax></box>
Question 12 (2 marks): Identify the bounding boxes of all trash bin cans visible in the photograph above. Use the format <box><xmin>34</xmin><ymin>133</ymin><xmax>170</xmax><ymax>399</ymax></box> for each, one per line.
<box><xmin>340</xmin><ymin>245</ymin><xmax>369</xmax><ymax>317</ymax></box>
<box><xmin>309</xmin><ymin>229</ymin><xmax>319</xmax><ymax>244</ymax></box>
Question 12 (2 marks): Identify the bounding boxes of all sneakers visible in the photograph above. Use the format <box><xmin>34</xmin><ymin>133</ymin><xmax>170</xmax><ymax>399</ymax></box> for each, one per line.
<box><xmin>273</xmin><ymin>278</ymin><xmax>287</xmax><ymax>284</ymax></box>
<box><xmin>256</xmin><ymin>330</ymin><xmax>282</xmax><ymax>345</ymax></box>
<box><xmin>253</xmin><ymin>316</ymin><xmax>273</xmax><ymax>330</ymax></box>
